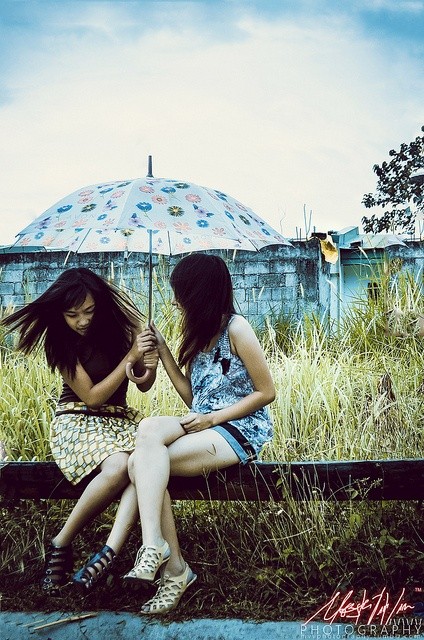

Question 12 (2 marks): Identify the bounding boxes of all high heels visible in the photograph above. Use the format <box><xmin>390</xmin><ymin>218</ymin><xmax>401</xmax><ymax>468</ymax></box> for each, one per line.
<box><xmin>72</xmin><ymin>544</ymin><xmax>118</xmax><ymax>598</ymax></box>
<box><xmin>138</xmin><ymin>562</ymin><xmax>197</xmax><ymax>615</ymax></box>
<box><xmin>122</xmin><ymin>539</ymin><xmax>171</xmax><ymax>588</ymax></box>
<box><xmin>38</xmin><ymin>541</ymin><xmax>75</xmax><ymax>600</ymax></box>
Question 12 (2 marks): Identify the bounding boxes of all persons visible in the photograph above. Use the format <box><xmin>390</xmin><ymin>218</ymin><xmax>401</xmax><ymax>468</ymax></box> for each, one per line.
<box><xmin>0</xmin><ymin>266</ymin><xmax>160</xmax><ymax>600</ymax></box>
<box><xmin>123</xmin><ymin>252</ymin><xmax>277</xmax><ymax>616</ymax></box>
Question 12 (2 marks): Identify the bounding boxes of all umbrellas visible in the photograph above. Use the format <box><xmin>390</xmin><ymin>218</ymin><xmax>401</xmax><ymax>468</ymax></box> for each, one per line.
<box><xmin>8</xmin><ymin>155</ymin><xmax>297</xmax><ymax>386</ymax></box>
